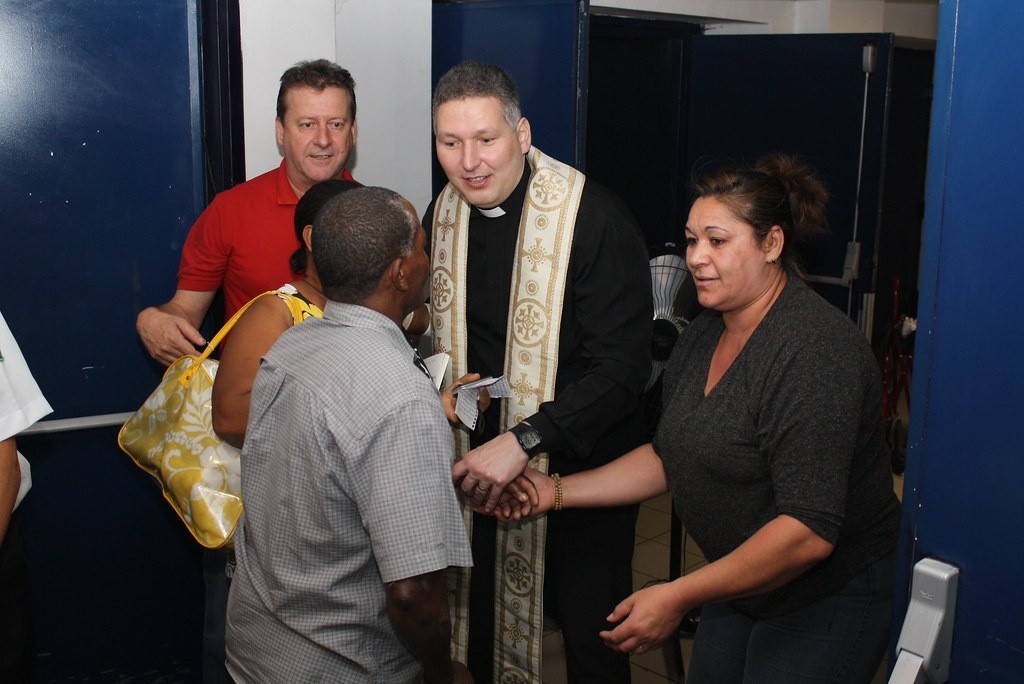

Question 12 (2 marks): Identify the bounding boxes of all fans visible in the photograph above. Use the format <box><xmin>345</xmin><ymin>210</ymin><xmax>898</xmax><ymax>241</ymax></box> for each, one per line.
<box><xmin>638</xmin><ymin>247</ymin><xmax>705</xmax><ymax>639</ymax></box>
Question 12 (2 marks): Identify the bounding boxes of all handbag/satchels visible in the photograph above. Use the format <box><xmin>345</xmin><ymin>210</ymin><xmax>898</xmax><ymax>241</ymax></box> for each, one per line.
<box><xmin>118</xmin><ymin>291</ymin><xmax>302</xmax><ymax>549</ymax></box>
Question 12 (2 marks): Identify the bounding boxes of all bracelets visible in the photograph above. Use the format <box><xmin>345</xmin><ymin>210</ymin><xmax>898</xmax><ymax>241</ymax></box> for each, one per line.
<box><xmin>551</xmin><ymin>472</ymin><xmax>563</xmax><ymax>511</ymax></box>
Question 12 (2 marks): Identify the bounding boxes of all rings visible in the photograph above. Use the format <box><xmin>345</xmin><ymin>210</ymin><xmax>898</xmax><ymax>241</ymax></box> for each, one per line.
<box><xmin>478</xmin><ymin>485</ymin><xmax>487</xmax><ymax>491</ymax></box>
<box><xmin>639</xmin><ymin>641</ymin><xmax>644</xmax><ymax>648</ymax></box>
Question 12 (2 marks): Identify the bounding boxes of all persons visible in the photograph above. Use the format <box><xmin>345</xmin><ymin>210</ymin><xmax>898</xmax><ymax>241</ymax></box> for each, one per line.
<box><xmin>471</xmin><ymin>149</ymin><xmax>900</xmax><ymax>684</ymax></box>
<box><xmin>133</xmin><ymin>59</ymin><xmax>367</xmax><ymax>368</ymax></box>
<box><xmin>420</xmin><ymin>62</ymin><xmax>649</xmax><ymax>684</ymax></box>
<box><xmin>222</xmin><ymin>186</ymin><xmax>474</xmax><ymax>684</ymax></box>
<box><xmin>201</xmin><ymin>178</ymin><xmax>538</xmax><ymax>684</ymax></box>
<box><xmin>0</xmin><ymin>311</ymin><xmax>54</xmax><ymax>541</ymax></box>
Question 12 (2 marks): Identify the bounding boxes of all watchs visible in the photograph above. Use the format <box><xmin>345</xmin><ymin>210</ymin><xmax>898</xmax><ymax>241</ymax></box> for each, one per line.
<box><xmin>508</xmin><ymin>422</ymin><xmax>543</xmax><ymax>460</ymax></box>
